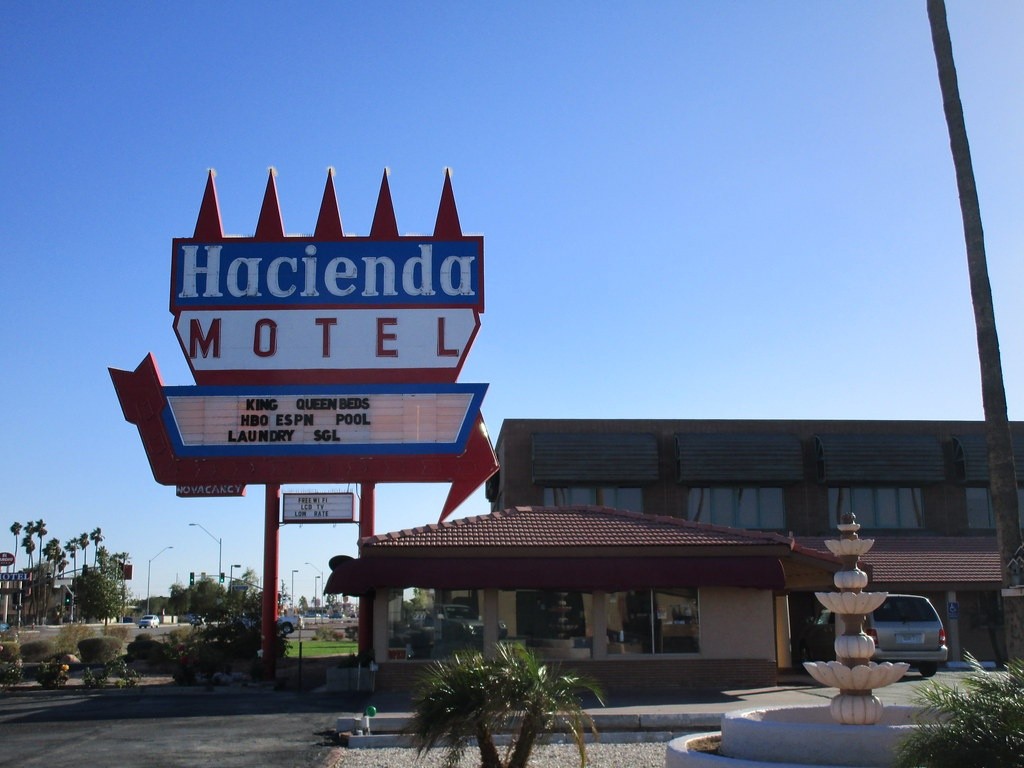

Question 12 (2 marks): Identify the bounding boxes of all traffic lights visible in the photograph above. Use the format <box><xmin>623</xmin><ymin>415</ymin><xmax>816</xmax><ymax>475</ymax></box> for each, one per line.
<box><xmin>218</xmin><ymin>572</ymin><xmax>224</xmax><ymax>584</ymax></box>
<box><xmin>189</xmin><ymin>572</ymin><xmax>196</xmax><ymax>585</ymax></box>
<box><xmin>64</xmin><ymin>592</ymin><xmax>71</xmax><ymax>606</ymax></box>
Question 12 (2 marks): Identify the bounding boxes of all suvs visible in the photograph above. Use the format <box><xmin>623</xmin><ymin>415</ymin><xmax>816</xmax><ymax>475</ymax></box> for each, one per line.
<box><xmin>796</xmin><ymin>595</ymin><xmax>948</xmax><ymax>678</ymax></box>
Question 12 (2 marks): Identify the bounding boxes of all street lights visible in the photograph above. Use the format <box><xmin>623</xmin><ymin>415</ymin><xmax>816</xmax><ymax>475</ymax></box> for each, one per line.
<box><xmin>229</xmin><ymin>564</ymin><xmax>242</xmax><ymax>623</ymax></box>
<box><xmin>315</xmin><ymin>576</ymin><xmax>320</xmax><ymax>617</ymax></box>
<box><xmin>148</xmin><ymin>546</ymin><xmax>173</xmax><ymax>614</ymax></box>
<box><xmin>190</xmin><ymin>523</ymin><xmax>222</xmax><ymax>584</ymax></box>
<box><xmin>291</xmin><ymin>570</ymin><xmax>298</xmax><ymax>614</ymax></box>
<box><xmin>305</xmin><ymin>563</ymin><xmax>323</xmax><ymax>625</ymax></box>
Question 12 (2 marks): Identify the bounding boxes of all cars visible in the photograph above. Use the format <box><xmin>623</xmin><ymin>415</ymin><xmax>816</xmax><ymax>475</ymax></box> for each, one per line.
<box><xmin>1</xmin><ymin>620</ymin><xmax>10</xmax><ymax>634</ymax></box>
<box><xmin>190</xmin><ymin>617</ymin><xmax>202</xmax><ymax>626</ymax></box>
<box><xmin>327</xmin><ymin>612</ymin><xmax>342</xmax><ymax>619</ymax></box>
<box><xmin>305</xmin><ymin>611</ymin><xmax>320</xmax><ymax>618</ymax></box>
<box><xmin>277</xmin><ymin>616</ymin><xmax>303</xmax><ymax>634</ymax></box>
<box><xmin>438</xmin><ymin>605</ymin><xmax>507</xmax><ymax>643</ymax></box>
<box><xmin>139</xmin><ymin>616</ymin><xmax>160</xmax><ymax>628</ymax></box>
